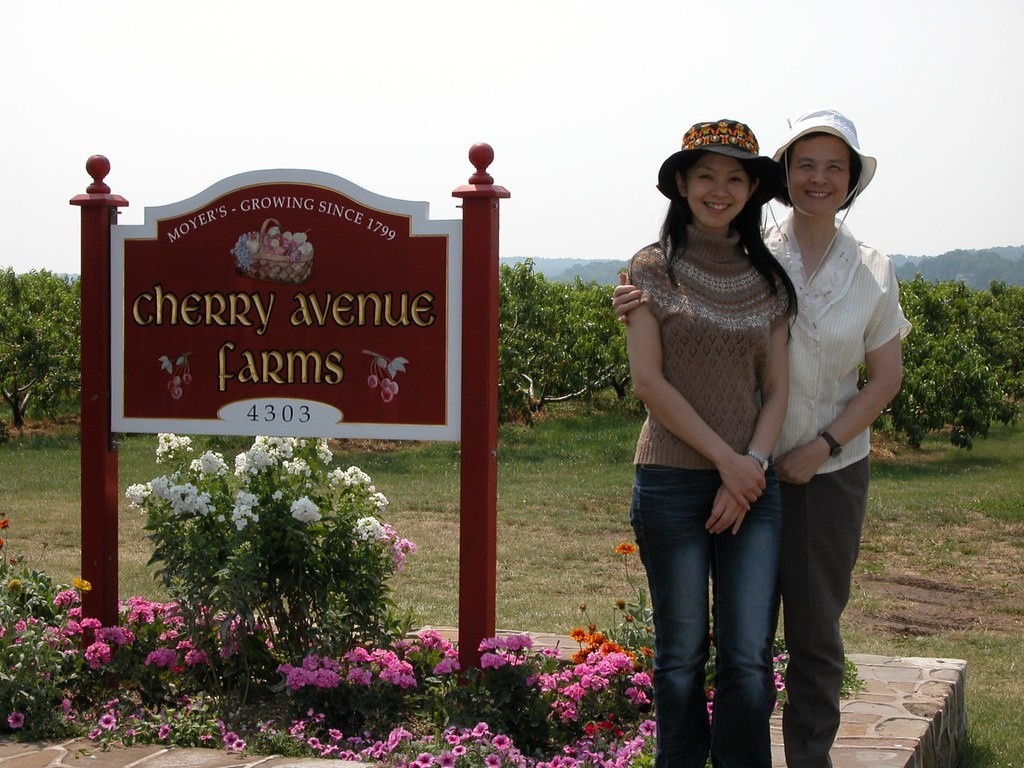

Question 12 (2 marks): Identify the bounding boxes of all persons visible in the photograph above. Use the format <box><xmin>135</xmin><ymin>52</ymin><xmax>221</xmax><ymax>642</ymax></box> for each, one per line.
<box><xmin>625</xmin><ymin>121</ymin><xmax>799</xmax><ymax>768</ymax></box>
<box><xmin>613</xmin><ymin>109</ymin><xmax>912</xmax><ymax>768</ymax></box>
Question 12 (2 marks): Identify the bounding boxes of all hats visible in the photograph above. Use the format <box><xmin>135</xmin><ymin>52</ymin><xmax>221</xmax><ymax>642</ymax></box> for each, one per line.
<box><xmin>657</xmin><ymin>118</ymin><xmax>778</xmax><ymax>200</ymax></box>
<box><xmin>772</xmin><ymin>110</ymin><xmax>877</xmax><ymax>196</ymax></box>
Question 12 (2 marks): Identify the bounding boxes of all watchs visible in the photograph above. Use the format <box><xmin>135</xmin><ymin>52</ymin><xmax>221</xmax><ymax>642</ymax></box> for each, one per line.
<box><xmin>819</xmin><ymin>430</ymin><xmax>842</xmax><ymax>457</ymax></box>
<box><xmin>748</xmin><ymin>450</ymin><xmax>768</xmax><ymax>470</ymax></box>
